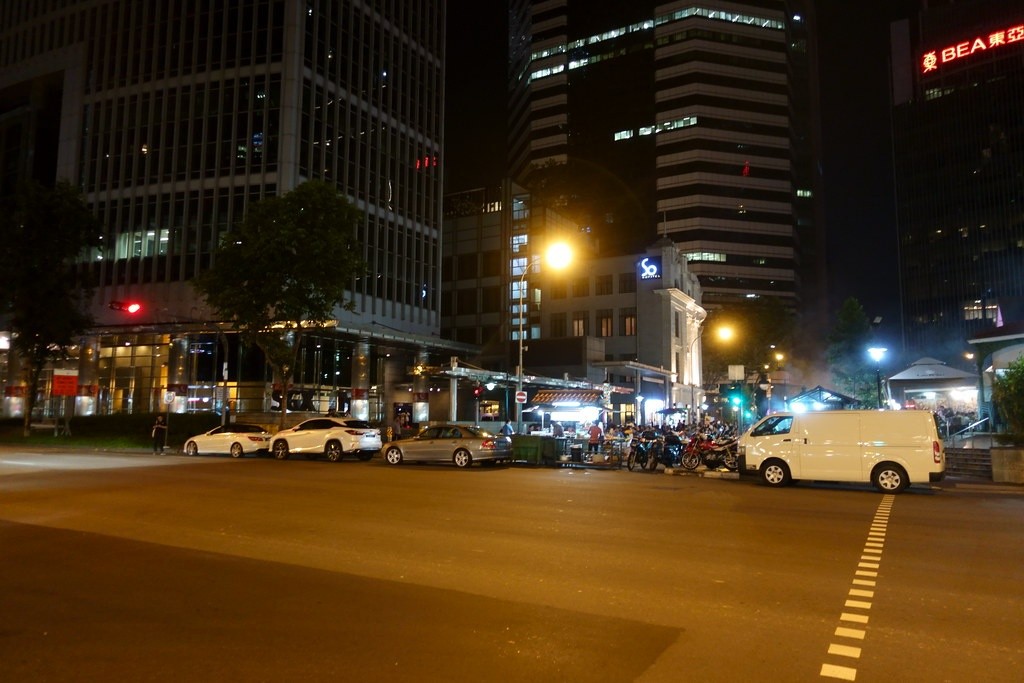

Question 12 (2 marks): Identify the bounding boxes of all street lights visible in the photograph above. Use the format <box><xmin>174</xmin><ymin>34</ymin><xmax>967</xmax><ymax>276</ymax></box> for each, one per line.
<box><xmin>690</xmin><ymin>327</ymin><xmax>731</xmax><ymax>424</ymax></box>
<box><xmin>867</xmin><ymin>345</ymin><xmax>888</xmax><ymax>409</ymax></box>
<box><xmin>517</xmin><ymin>242</ymin><xmax>575</xmax><ymax>433</ymax></box>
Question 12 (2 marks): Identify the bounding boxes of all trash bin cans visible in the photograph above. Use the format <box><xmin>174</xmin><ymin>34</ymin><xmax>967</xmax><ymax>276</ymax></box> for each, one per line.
<box><xmin>511</xmin><ymin>434</ymin><xmax>557</xmax><ymax>463</ymax></box>
<box><xmin>569</xmin><ymin>445</ymin><xmax>582</xmax><ymax>462</ymax></box>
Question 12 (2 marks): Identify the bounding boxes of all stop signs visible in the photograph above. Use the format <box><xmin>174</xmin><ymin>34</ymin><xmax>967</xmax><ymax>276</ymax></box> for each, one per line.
<box><xmin>515</xmin><ymin>391</ymin><xmax>527</xmax><ymax>403</ymax></box>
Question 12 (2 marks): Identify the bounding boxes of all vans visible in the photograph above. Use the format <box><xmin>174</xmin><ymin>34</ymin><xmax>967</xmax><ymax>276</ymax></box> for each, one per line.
<box><xmin>738</xmin><ymin>410</ymin><xmax>945</xmax><ymax>493</ymax></box>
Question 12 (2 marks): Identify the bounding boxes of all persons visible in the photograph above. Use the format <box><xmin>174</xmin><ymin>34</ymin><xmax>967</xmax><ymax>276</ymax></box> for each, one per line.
<box><xmin>526</xmin><ymin>425</ymin><xmax>540</xmax><ymax>434</ymax></box>
<box><xmin>153</xmin><ymin>415</ymin><xmax>168</xmax><ymax>456</ymax></box>
<box><xmin>552</xmin><ymin>421</ymin><xmax>564</xmax><ymax>437</ymax></box>
<box><xmin>906</xmin><ymin>395</ymin><xmax>975</xmax><ymax>432</ymax></box>
<box><xmin>503</xmin><ymin>419</ymin><xmax>514</xmax><ymax>435</ymax></box>
<box><xmin>588</xmin><ymin>420</ymin><xmax>601</xmax><ymax>454</ymax></box>
<box><xmin>605</xmin><ymin>418</ymin><xmax>731</xmax><ymax>442</ymax></box>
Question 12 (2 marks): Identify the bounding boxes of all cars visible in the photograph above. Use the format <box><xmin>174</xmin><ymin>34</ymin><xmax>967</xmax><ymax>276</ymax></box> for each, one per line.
<box><xmin>380</xmin><ymin>424</ymin><xmax>514</xmax><ymax>469</ymax></box>
<box><xmin>183</xmin><ymin>423</ymin><xmax>273</xmax><ymax>458</ymax></box>
<box><xmin>268</xmin><ymin>417</ymin><xmax>382</xmax><ymax>463</ymax></box>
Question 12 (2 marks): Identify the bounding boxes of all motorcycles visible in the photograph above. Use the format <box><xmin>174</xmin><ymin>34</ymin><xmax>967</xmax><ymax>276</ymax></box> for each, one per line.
<box><xmin>627</xmin><ymin>431</ymin><xmax>738</xmax><ymax>470</ymax></box>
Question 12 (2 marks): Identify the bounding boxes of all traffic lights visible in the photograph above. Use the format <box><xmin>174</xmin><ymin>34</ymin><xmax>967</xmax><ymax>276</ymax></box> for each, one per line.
<box><xmin>108</xmin><ymin>301</ymin><xmax>142</xmax><ymax>313</ymax></box>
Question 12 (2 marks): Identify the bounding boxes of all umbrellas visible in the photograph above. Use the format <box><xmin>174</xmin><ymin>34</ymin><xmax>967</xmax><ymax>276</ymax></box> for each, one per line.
<box><xmin>655</xmin><ymin>408</ymin><xmax>686</xmax><ymax>419</ymax></box>
